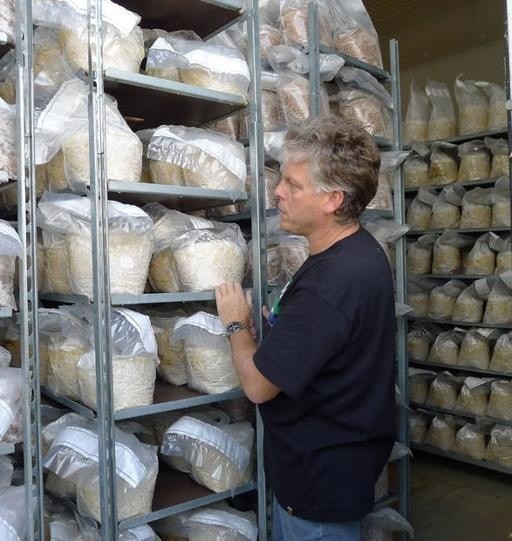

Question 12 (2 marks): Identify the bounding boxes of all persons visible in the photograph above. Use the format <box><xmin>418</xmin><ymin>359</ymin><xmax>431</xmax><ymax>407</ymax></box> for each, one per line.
<box><xmin>214</xmin><ymin>113</ymin><xmax>397</xmax><ymax>541</ymax></box>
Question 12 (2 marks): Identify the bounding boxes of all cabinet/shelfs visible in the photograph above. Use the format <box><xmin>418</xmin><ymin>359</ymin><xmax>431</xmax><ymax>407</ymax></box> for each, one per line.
<box><xmin>0</xmin><ymin>0</ymin><xmax>44</xmax><ymax>540</ymax></box>
<box><xmin>402</xmin><ymin>55</ymin><xmax>512</xmax><ymax>475</ymax></box>
<box><xmin>211</xmin><ymin>2</ymin><xmax>408</xmax><ymax>541</ymax></box>
<box><xmin>39</xmin><ymin>0</ymin><xmax>266</xmax><ymax>541</ymax></box>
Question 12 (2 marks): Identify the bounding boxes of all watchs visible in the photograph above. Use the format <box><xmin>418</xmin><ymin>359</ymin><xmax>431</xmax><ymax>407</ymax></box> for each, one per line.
<box><xmin>225</xmin><ymin>321</ymin><xmax>250</xmax><ymax>340</ymax></box>
